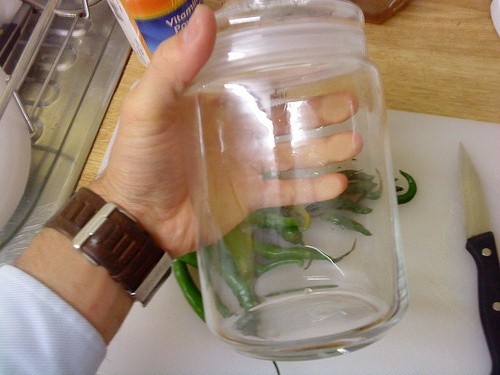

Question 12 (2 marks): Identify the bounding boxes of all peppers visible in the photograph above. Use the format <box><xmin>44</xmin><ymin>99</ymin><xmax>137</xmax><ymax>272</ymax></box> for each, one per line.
<box><xmin>172</xmin><ymin>168</ymin><xmax>416</xmax><ymax>327</ymax></box>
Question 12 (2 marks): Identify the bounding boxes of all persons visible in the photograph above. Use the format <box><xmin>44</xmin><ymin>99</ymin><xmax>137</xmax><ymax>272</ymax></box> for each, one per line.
<box><xmin>1</xmin><ymin>5</ymin><xmax>364</xmax><ymax>374</ymax></box>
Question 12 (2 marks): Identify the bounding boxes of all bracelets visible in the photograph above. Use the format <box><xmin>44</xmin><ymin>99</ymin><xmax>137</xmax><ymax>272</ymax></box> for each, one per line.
<box><xmin>43</xmin><ymin>187</ymin><xmax>173</xmax><ymax>307</ymax></box>
